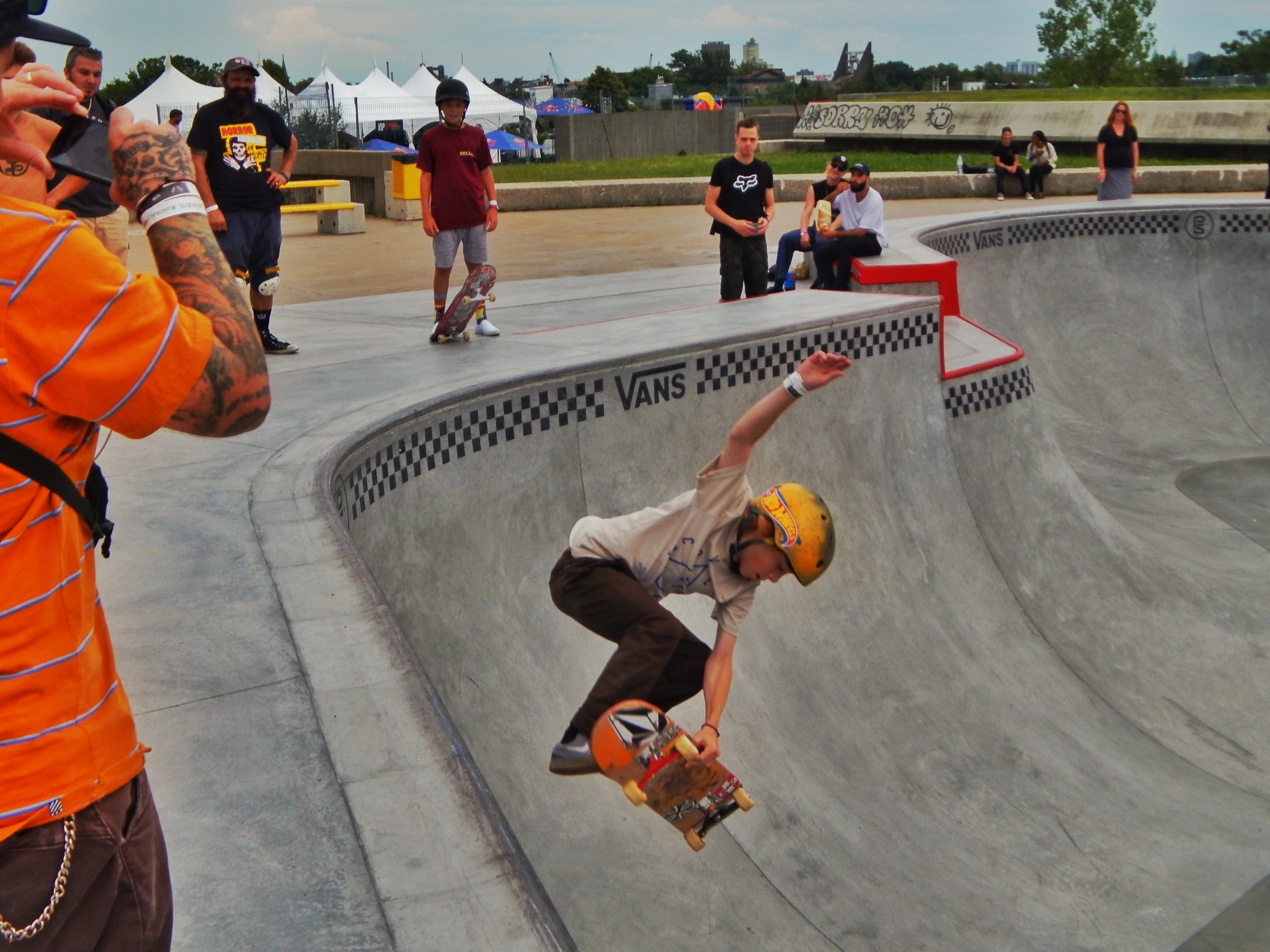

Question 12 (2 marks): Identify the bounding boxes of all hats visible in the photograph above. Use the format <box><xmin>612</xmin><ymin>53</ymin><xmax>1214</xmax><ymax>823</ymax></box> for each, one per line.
<box><xmin>220</xmin><ymin>57</ymin><xmax>261</xmax><ymax>78</ymax></box>
<box><xmin>849</xmin><ymin>162</ymin><xmax>870</xmax><ymax>175</ymax></box>
<box><xmin>0</xmin><ymin>0</ymin><xmax>91</xmax><ymax>48</ymax></box>
<box><xmin>831</xmin><ymin>154</ymin><xmax>848</xmax><ymax>169</ymax></box>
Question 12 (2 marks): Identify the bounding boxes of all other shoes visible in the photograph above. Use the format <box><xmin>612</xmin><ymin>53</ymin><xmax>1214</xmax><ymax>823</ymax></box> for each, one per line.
<box><xmin>475</xmin><ymin>318</ymin><xmax>501</xmax><ymax>337</ymax></box>
<box><xmin>997</xmin><ymin>193</ymin><xmax>1005</xmax><ymax>201</ymax></box>
<box><xmin>549</xmin><ymin>734</ymin><xmax>604</xmax><ymax>776</ymax></box>
<box><xmin>430</xmin><ymin>321</ymin><xmax>440</xmax><ymax>339</ymax></box>
<box><xmin>767</xmin><ymin>286</ymin><xmax>784</xmax><ymax>293</ymax></box>
<box><xmin>1025</xmin><ymin>192</ymin><xmax>1035</xmax><ymax>200</ymax></box>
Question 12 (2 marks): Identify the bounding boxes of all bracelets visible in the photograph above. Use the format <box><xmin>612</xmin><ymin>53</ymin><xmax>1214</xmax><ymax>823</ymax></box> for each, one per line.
<box><xmin>26</xmin><ymin>72</ymin><xmax>32</xmax><ymax>84</ymax></box>
<box><xmin>701</xmin><ymin>723</ymin><xmax>720</xmax><ymax>738</ymax></box>
<box><xmin>782</xmin><ymin>377</ymin><xmax>802</xmax><ymax>399</ymax></box>
<box><xmin>789</xmin><ymin>371</ymin><xmax>808</xmax><ymax>396</ymax></box>
<box><xmin>279</xmin><ymin>172</ymin><xmax>289</xmax><ymax>182</ymax></box>
<box><xmin>764</xmin><ymin>217</ymin><xmax>769</xmax><ymax>223</ymax></box>
<box><xmin>1099</xmin><ymin>169</ymin><xmax>1106</xmax><ymax>172</ymax></box>
<box><xmin>140</xmin><ymin>194</ymin><xmax>208</xmax><ymax>234</ymax></box>
<box><xmin>800</xmin><ymin>231</ymin><xmax>808</xmax><ymax>236</ymax></box>
<box><xmin>489</xmin><ymin>205</ymin><xmax>498</xmax><ymax>211</ymax></box>
<box><xmin>136</xmin><ymin>180</ymin><xmax>201</xmax><ymax>224</ymax></box>
<box><xmin>205</xmin><ymin>205</ymin><xmax>219</xmax><ymax>213</ymax></box>
<box><xmin>136</xmin><ymin>176</ymin><xmax>196</xmax><ymax>227</ymax></box>
<box><xmin>489</xmin><ymin>200</ymin><xmax>499</xmax><ymax>209</ymax></box>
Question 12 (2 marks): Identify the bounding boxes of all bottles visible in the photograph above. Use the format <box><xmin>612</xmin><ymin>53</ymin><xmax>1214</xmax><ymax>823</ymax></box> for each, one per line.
<box><xmin>784</xmin><ymin>263</ymin><xmax>796</xmax><ymax>291</ymax></box>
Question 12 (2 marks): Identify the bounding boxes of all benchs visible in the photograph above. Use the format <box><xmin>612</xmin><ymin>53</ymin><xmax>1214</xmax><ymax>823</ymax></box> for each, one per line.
<box><xmin>276</xmin><ymin>177</ymin><xmax>366</xmax><ymax>234</ymax></box>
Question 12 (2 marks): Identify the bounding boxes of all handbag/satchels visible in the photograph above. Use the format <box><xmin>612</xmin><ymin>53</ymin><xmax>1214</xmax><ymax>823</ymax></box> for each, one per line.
<box><xmin>962</xmin><ymin>162</ymin><xmax>987</xmax><ymax>173</ymax></box>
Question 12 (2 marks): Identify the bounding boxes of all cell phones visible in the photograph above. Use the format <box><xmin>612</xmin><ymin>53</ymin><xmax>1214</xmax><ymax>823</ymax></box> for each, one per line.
<box><xmin>749</xmin><ymin>223</ymin><xmax>761</xmax><ymax>235</ymax></box>
<box><xmin>38</xmin><ymin>109</ymin><xmax>116</xmax><ymax>186</ymax></box>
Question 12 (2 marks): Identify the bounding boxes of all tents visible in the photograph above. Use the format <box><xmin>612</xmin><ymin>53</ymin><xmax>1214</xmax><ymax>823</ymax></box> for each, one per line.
<box><xmin>123</xmin><ymin>54</ymin><xmax>542</xmax><ymax>163</ymax></box>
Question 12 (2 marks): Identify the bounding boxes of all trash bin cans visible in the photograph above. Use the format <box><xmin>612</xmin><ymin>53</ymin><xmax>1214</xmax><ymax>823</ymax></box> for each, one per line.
<box><xmin>392</xmin><ymin>152</ymin><xmax>421</xmax><ymax>201</ymax></box>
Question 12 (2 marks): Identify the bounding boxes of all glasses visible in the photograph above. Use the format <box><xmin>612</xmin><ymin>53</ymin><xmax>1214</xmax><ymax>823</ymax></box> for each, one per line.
<box><xmin>29</xmin><ymin>0</ymin><xmax>48</xmax><ymax>15</ymax></box>
<box><xmin>831</xmin><ymin>163</ymin><xmax>845</xmax><ymax>172</ymax></box>
<box><xmin>1114</xmin><ymin>109</ymin><xmax>1127</xmax><ymax>114</ymax></box>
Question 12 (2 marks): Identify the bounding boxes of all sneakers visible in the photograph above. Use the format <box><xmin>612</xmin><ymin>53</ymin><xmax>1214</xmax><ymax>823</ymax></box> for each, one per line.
<box><xmin>259</xmin><ymin>331</ymin><xmax>299</xmax><ymax>354</ymax></box>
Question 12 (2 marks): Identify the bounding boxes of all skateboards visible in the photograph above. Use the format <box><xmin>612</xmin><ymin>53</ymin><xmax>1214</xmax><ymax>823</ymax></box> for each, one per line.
<box><xmin>429</xmin><ymin>264</ymin><xmax>497</xmax><ymax>345</ymax></box>
<box><xmin>588</xmin><ymin>698</ymin><xmax>755</xmax><ymax>853</ymax></box>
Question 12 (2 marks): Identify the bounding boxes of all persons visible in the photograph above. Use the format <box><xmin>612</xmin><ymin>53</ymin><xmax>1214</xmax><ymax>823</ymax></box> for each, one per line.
<box><xmin>818</xmin><ymin>163</ymin><xmax>882</xmax><ymax>291</ymax></box>
<box><xmin>159</xmin><ymin>109</ymin><xmax>183</xmax><ymax>138</ymax></box>
<box><xmin>1096</xmin><ymin>101</ymin><xmax>1138</xmax><ymax>200</ymax></box>
<box><xmin>0</xmin><ymin>0</ymin><xmax>270</xmax><ymax>952</ymax></box>
<box><xmin>545</xmin><ymin>351</ymin><xmax>854</xmax><ymax>777</ymax></box>
<box><xmin>1025</xmin><ymin>130</ymin><xmax>1058</xmax><ymax>198</ymax></box>
<box><xmin>766</xmin><ymin>154</ymin><xmax>850</xmax><ymax>293</ymax></box>
<box><xmin>704</xmin><ymin>117</ymin><xmax>776</xmax><ymax>301</ymax></box>
<box><xmin>417</xmin><ymin>79</ymin><xmax>502</xmax><ymax>336</ymax></box>
<box><xmin>991</xmin><ymin>126</ymin><xmax>1034</xmax><ymax>201</ymax></box>
<box><xmin>184</xmin><ymin>57</ymin><xmax>299</xmax><ymax>354</ymax></box>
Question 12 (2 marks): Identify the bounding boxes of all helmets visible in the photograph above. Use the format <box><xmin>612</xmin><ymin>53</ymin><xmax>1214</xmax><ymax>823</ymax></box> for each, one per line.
<box><xmin>747</xmin><ymin>482</ymin><xmax>837</xmax><ymax>589</ymax></box>
<box><xmin>435</xmin><ymin>78</ymin><xmax>470</xmax><ymax>103</ymax></box>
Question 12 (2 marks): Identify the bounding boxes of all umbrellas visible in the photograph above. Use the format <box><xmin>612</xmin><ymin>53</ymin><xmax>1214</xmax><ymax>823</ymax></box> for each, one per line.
<box><xmin>532</xmin><ymin>98</ymin><xmax>595</xmax><ymax>116</ymax></box>
<box><xmin>483</xmin><ymin>129</ymin><xmax>548</xmax><ymax>150</ymax></box>
